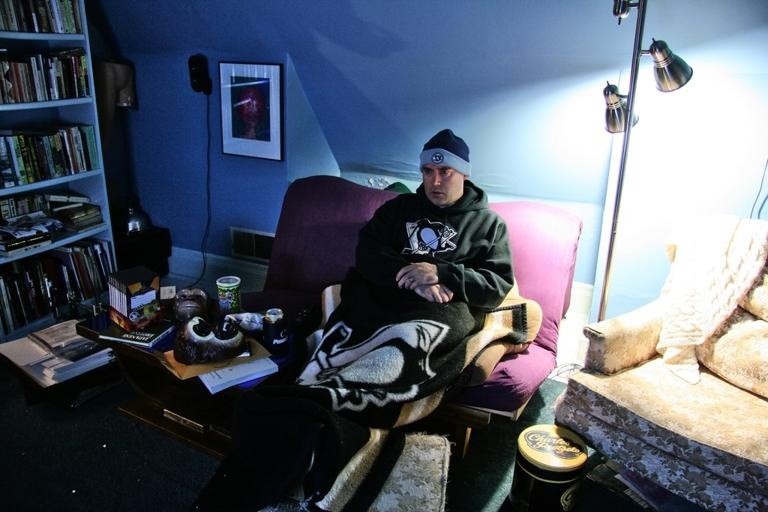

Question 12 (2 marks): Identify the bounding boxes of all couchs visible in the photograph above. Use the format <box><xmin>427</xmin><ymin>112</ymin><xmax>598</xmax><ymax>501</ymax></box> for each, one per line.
<box><xmin>257</xmin><ymin>174</ymin><xmax>583</xmax><ymax>463</ymax></box>
<box><xmin>552</xmin><ymin>216</ymin><xmax>767</xmax><ymax>512</ymax></box>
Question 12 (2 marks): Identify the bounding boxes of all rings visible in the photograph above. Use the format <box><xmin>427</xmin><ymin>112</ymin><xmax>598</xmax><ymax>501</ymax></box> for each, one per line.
<box><xmin>409</xmin><ymin>277</ymin><xmax>415</xmax><ymax>282</ymax></box>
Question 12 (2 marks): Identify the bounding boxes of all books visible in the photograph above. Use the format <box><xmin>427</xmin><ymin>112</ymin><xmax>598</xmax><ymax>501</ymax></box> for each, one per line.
<box><xmin>0</xmin><ymin>189</ymin><xmax>103</xmax><ymax>257</ymax></box>
<box><xmin>0</xmin><ymin>123</ymin><xmax>100</xmax><ymax>189</ymax></box>
<box><xmin>0</xmin><ymin>319</ymin><xmax>116</xmax><ymax>388</ymax></box>
<box><xmin>0</xmin><ymin>0</ymin><xmax>83</xmax><ymax>33</ymax></box>
<box><xmin>0</xmin><ymin>47</ymin><xmax>91</xmax><ymax>104</ymax></box>
<box><xmin>99</xmin><ymin>320</ymin><xmax>175</xmax><ymax>349</ymax></box>
<box><xmin>0</xmin><ymin>238</ymin><xmax>116</xmax><ymax>335</ymax></box>
<box><xmin>108</xmin><ymin>265</ymin><xmax>160</xmax><ymax>333</ymax></box>
<box><xmin>197</xmin><ymin>345</ymin><xmax>279</xmax><ymax>394</ymax></box>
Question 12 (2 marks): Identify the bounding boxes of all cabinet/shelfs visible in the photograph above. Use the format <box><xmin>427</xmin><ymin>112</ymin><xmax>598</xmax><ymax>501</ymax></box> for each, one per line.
<box><xmin>0</xmin><ymin>0</ymin><xmax>122</xmax><ymax>340</ymax></box>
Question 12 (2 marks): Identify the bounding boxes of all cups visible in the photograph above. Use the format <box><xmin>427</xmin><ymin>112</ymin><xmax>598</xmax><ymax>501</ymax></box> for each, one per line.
<box><xmin>215</xmin><ymin>276</ymin><xmax>241</xmax><ymax>316</ymax></box>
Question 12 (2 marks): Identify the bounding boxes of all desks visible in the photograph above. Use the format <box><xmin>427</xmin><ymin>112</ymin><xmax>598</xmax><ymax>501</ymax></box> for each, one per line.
<box><xmin>74</xmin><ymin>298</ymin><xmax>293</xmax><ymax>468</ymax></box>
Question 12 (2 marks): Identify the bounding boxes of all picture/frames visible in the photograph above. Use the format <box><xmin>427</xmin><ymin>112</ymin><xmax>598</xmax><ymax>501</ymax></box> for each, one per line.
<box><xmin>218</xmin><ymin>61</ymin><xmax>284</xmax><ymax>163</ymax></box>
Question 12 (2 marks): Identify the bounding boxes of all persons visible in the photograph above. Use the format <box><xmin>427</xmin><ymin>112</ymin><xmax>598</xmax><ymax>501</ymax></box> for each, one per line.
<box><xmin>282</xmin><ymin>129</ymin><xmax>514</xmax><ymax>504</ymax></box>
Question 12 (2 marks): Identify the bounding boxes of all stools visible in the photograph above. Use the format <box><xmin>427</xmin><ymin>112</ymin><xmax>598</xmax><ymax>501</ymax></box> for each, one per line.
<box><xmin>509</xmin><ymin>424</ymin><xmax>588</xmax><ymax>512</ymax></box>
<box><xmin>254</xmin><ymin>434</ymin><xmax>452</xmax><ymax>511</ymax></box>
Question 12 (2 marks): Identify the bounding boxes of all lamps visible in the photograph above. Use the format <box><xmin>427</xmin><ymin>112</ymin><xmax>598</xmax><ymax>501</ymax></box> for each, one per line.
<box><xmin>596</xmin><ymin>0</ymin><xmax>693</xmax><ymax>325</ymax></box>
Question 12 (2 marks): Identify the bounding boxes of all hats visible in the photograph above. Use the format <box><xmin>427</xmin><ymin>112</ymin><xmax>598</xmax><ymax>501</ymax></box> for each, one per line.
<box><xmin>418</xmin><ymin>127</ymin><xmax>472</xmax><ymax>177</ymax></box>
